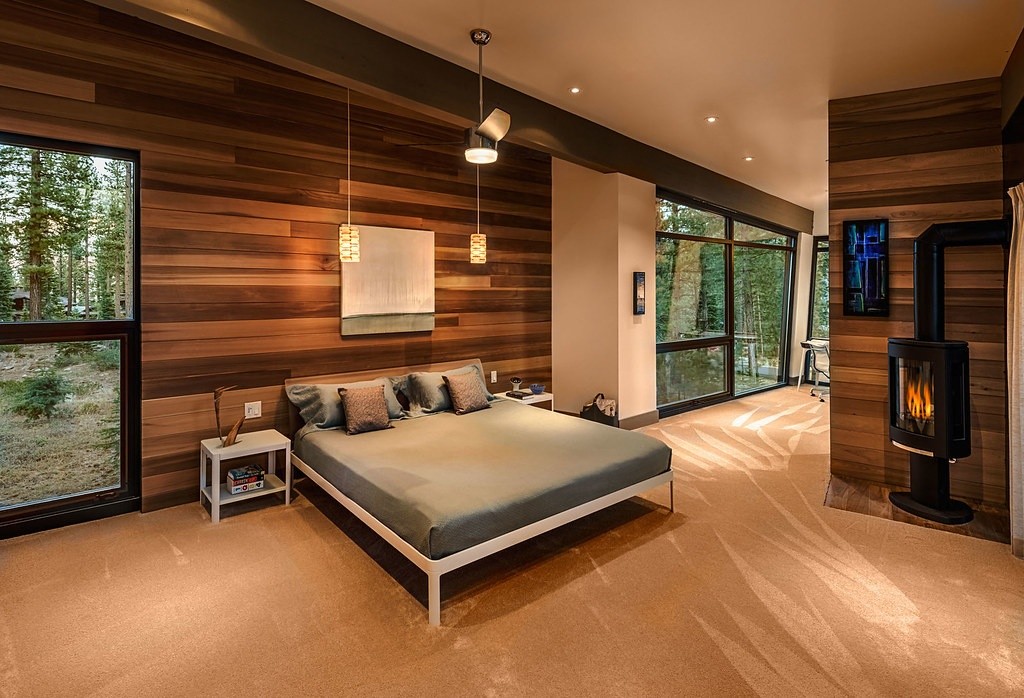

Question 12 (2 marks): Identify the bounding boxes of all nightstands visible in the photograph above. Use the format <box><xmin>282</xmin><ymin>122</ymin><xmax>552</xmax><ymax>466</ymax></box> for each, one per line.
<box><xmin>200</xmin><ymin>428</ymin><xmax>291</xmax><ymax>523</ymax></box>
<box><xmin>493</xmin><ymin>388</ymin><xmax>554</xmax><ymax>412</ymax></box>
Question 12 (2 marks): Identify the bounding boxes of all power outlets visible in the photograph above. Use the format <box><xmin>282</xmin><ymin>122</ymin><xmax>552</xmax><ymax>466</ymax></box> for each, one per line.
<box><xmin>245</xmin><ymin>401</ymin><xmax>262</xmax><ymax>420</ymax></box>
<box><xmin>491</xmin><ymin>371</ymin><xmax>497</xmax><ymax>383</ymax></box>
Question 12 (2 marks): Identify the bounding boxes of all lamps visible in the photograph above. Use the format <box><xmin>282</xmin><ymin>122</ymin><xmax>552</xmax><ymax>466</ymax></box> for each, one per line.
<box><xmin>339</xmin><ymin>88</ymin><xmax>360</xmax><ymax>262</ymax></box>
<box><xmin>470</xmin><ymin>164</ymin><xmax>486</xmax><ymax>264</ymax></box>
<box><xmin>397</xmin><ymin>28</ymin><xmax>511</xmax><ymax>163</ymax></box>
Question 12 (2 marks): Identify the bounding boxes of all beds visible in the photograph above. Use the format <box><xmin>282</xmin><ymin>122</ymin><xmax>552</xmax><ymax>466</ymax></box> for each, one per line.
<box><xmin>290</xmin><ymin>394</ymin><xmax>673</xmax><ymax>625</ymax></box>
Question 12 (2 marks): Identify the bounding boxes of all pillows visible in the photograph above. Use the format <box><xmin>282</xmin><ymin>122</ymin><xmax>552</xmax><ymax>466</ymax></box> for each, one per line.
<box><xmin>441</xmin><ymin>370</ymin><xmax>492</xmax><ymax>415</ymax></box>
<box><xmin>337</xmin><ymin>385</ymin><xmax>396</xmax><ymax>435</ymax></box>
<box><xmin>284</xmin><ymin>377</ymin><xmax>410</xmax><ymax>439</ymax></box>
<box><xmin>390</xmin><ymin>362</ymin><xmax>506</xmax><ymax>419</ymax></box>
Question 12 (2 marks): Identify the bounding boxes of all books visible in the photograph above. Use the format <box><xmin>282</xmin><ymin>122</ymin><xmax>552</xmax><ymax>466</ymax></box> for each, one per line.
<box><xmin>226</xmin><ymin>463</ymin><xmax>266</xmax><ymax>495</ymax></box>
<box><xmin>506</xmin><ymin>391</ymin><xmax>535</xmax><ymax>400</ymax></box>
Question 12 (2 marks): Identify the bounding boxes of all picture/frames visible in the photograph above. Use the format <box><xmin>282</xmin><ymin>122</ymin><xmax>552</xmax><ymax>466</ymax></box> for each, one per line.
<box><xmin>633</xmin><ymin>271</ymin><xmax>645</xmax><ymax>315</ymax></box>
<box><xmin>843</xmin><ymin>219</ymin><xmax>890</xmax><ymax>317</ymax></box>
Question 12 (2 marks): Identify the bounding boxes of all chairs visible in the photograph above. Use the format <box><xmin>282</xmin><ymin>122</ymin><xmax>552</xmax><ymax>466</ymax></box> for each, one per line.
<box><xmin>800</xmin><ymin>342</ymin><xmax>829</xmax><ymax>402</ymax></box>
